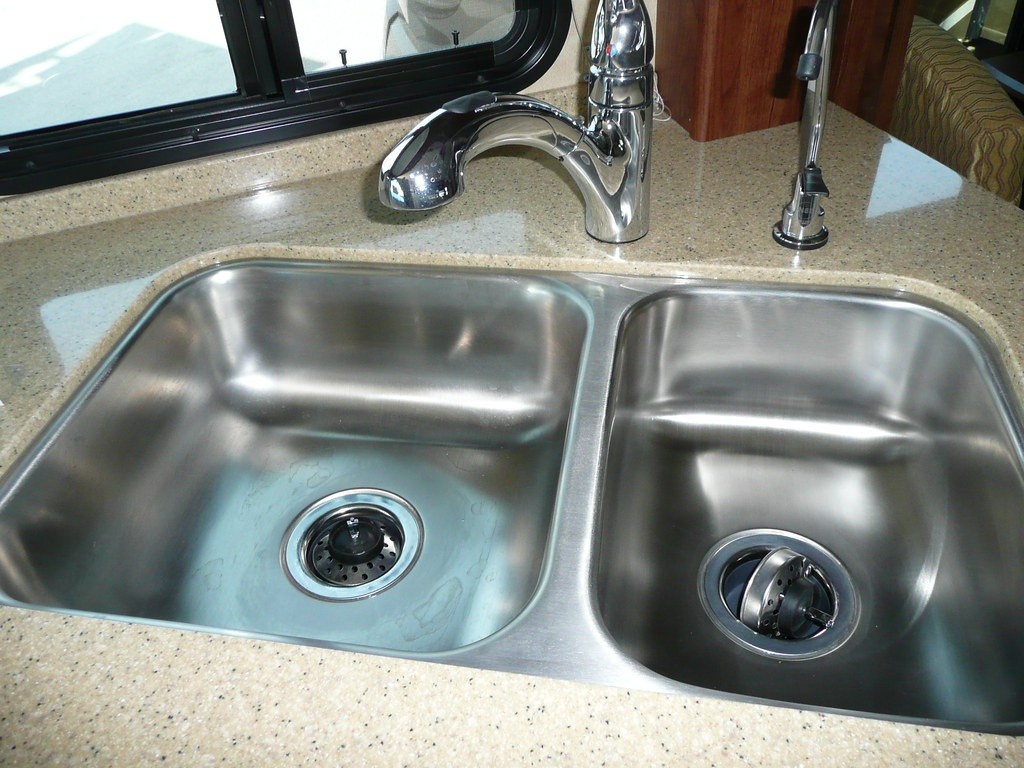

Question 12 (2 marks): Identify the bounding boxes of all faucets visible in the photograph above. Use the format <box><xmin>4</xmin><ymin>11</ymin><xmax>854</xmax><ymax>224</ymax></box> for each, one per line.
<box><xmin>765</xmin><ymin>0</ymin><xmax>842</xmax><ymax>252</ymax></box>
<box><xmin>377</xmin><ymin>0</ymin><xmax>656</xmax><ymax>246</ymax></box>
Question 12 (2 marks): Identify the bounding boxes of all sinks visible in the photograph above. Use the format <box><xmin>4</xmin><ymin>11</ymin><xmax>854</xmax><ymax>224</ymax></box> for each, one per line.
<box><xmin>0</xmin><ymin>254</ymin><xmax>595</xmax><ymax>682</ymax></box>
<box><xmin>587</xmin><ymin>280</ymin><xmax>1024</xmax><ymax>739</ymax></box>
